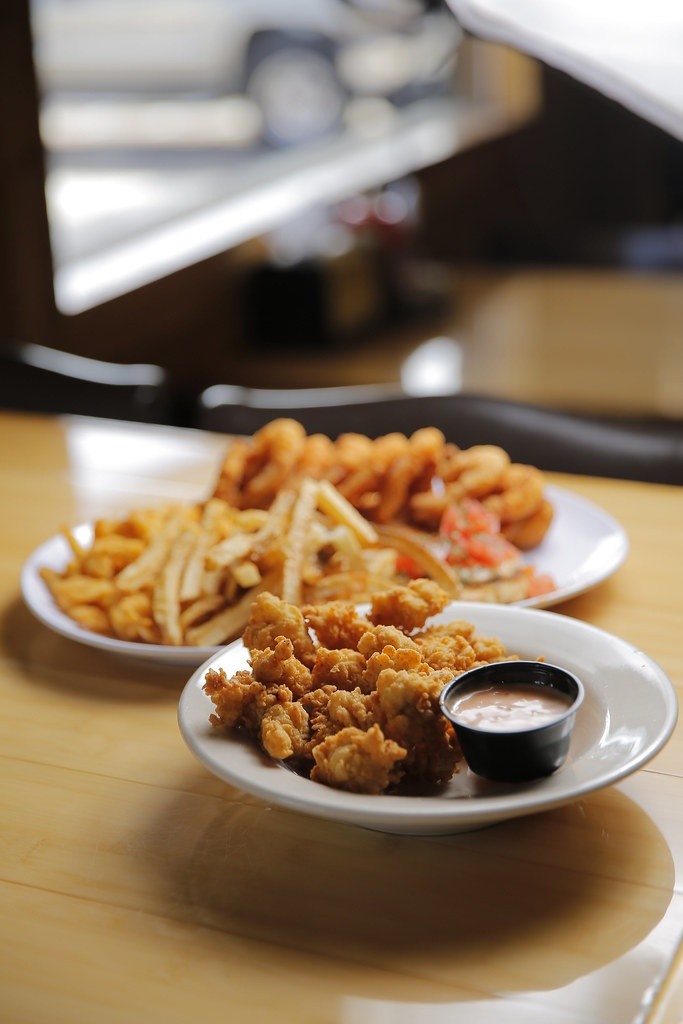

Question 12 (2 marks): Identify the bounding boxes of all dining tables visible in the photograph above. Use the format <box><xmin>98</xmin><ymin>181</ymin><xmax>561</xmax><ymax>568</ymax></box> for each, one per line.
<box><xmin>0</xmin><ymin>405</ymin><xmax>683</xmax><ymax>1024</ymax></box>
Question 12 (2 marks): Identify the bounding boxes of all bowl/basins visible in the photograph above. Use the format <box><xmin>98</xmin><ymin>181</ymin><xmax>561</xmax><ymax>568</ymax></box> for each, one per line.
<box><xmin>442</xmin><ymin>659</ymin><xmax>587</xmax><ymax>781</ymax></box>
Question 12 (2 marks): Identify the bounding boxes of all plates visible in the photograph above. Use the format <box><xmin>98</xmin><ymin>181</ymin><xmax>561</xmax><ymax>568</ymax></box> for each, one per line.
<box><xmin>19</xmin><ymin>480</ymin><xmax>631</xmax><ymax>669</ymax></box>
<box><xmin>177</xmin><ymin>600</ymin><xmax>679</xmax><ymax>838</ymax></box>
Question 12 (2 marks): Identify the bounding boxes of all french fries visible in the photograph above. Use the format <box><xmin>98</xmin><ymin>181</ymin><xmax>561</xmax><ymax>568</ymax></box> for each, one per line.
<box><xmin>36</xmin><ymin>476</ymin><xmax>460</xmax><ymax>648</ymax></box>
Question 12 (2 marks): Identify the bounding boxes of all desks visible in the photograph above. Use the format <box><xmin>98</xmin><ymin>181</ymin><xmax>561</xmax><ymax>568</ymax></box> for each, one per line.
<box><xmin>124</xmin><ymin>268</ymin><xmax>683</xmax><ymax>480</ymax></box>
